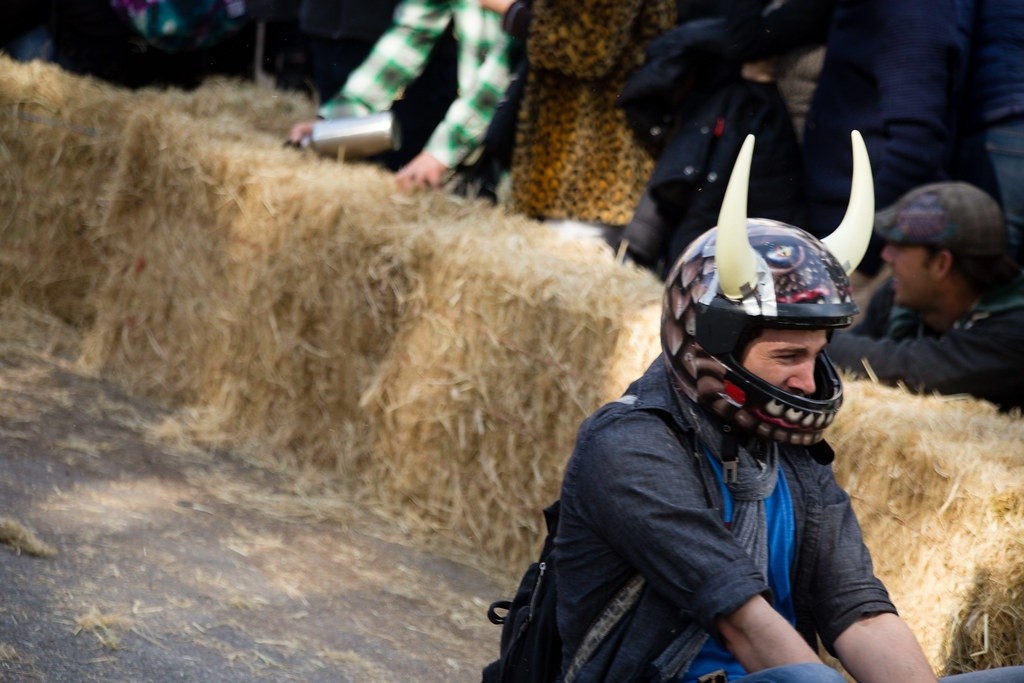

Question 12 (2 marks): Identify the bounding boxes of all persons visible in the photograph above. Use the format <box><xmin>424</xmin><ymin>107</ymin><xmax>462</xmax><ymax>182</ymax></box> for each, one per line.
<box><xmin>614</xmin><ymin>0</ymin><xmax>1024</xmax><ymax>287</ymax></box>
<box><xmin>292</xmin><ymin>0</ymin><xmax>520</xmax><ymax>203</ymax></box>
<box><xmin>829</xmin><ymin>181</ymin><xmax>1024</xmax><ymax>410</ymax></box>
<box><xmin>489</xmin><ymin>130</ymin><xmax>937</xmax><ymax>683</ymax></box>
<box><xmin>480</xmin><ymin>0</ymin><xmax>682</xmax><ymax>233</ymax></box>
<box><xmin>0</xmin><ymin>0</ymin><xmax>459</xmax><ymax>170</ymax></box>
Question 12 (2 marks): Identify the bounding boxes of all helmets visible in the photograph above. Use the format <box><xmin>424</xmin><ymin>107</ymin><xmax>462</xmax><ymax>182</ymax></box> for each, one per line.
<box><xmin>660</xmin><ymin>219</ymin><xmax>860</xmax><ymax>444</ymax></box>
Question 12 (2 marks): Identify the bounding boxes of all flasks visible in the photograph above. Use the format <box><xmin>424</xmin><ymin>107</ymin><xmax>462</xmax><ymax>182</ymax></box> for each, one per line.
<box><xmin>289</xmin><ymin>111</ymin><xmax>401</xmax><ymax>157</ymax></box>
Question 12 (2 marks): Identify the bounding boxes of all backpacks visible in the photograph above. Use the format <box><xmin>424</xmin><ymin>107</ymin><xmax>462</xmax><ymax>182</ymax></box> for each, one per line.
<box><xmin>484</xmin><ymin>501</ymin><xmax>559</xmax><ymax>683</ymax></box>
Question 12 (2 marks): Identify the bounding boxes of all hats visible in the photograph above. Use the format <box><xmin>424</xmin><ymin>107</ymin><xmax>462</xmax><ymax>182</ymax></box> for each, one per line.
<box><xmin>873</xmin><ymin>181</ymin><xmax>1002</xmax><ymax>250</ymax></box>
